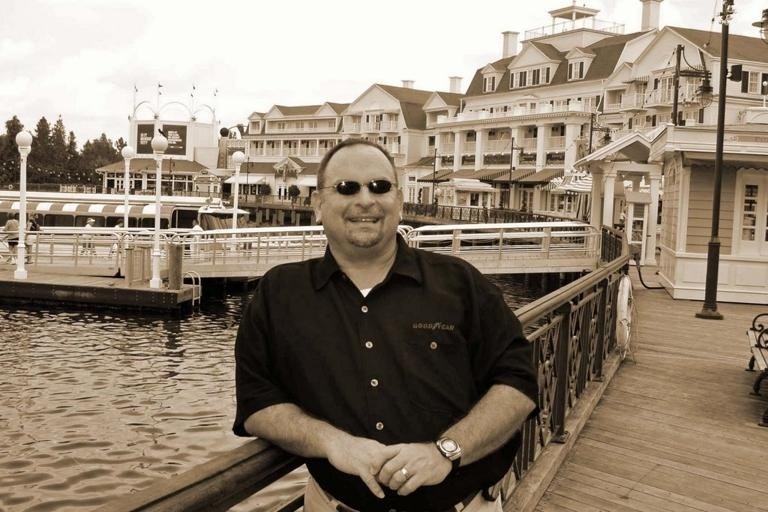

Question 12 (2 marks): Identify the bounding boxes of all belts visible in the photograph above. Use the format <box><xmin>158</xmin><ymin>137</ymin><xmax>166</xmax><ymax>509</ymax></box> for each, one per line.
<box><xmin>327</xmin><ymin>491</ymin><xmax>479</xmax><ymax>511</ymax></box>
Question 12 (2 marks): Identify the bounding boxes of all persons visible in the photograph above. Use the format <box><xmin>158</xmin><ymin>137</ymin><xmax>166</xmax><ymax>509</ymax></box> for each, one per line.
<box><xmin>620</xmin><ymin>210</ymin><xmax>626</xmax><ymax>223</ymax></box>
<box><xmin>3</xmin><ymin>211</ymin><xmax>19</xmax><ymax>264</ymax></box>
<box><xmin>111</xmin><ymin>219</ymin><xmax>124</xmax><ymax>259</ymax></box>
<box><xmin>23</xmin><ymin>211</ymin><xmax>39</xmax><ymax>265</ymax></box>
<box><xmin>186</xmin><ymin>219</ymin><xmax>203</xmax><ymax>258</ymax></box>
<box><xmin>235</xmin><ymin>138</ymin><xmax>537</xmax><ymax>510</ymax></box>
<box><xmin>80</xmin><ymin>217</ymin><xmax>100</xmax><ymax>256</ymax></box>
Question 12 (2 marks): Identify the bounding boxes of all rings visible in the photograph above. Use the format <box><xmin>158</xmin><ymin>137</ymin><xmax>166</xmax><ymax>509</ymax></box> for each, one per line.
<box><xmin>402</xmin><ymin>468</ymin><xmax>410</xmax><ymax>478</ymax></box>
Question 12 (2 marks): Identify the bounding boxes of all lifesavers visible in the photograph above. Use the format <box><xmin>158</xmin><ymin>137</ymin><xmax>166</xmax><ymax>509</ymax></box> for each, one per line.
<box><xmin>616</xmin><ymin>276</ymin><xmax>630</xmax><ymax>349</ymax></box>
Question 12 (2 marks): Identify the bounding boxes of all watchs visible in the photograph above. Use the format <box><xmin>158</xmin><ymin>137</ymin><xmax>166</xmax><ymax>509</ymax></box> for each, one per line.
<box><xmin>434</xmin><ymin>436</ymin><xmax>463</xmax><ymax>474</ymax></box>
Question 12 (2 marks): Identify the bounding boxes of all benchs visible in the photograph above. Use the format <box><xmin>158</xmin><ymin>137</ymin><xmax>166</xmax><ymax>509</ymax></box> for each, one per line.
<box><xmin>744</xmin><ymin>313</ymin><xmax>768</xmax><ymax>427</ymax></box>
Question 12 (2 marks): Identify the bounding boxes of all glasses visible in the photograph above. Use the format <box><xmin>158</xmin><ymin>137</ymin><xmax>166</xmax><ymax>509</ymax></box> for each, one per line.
<box><xmin>317</xmin><ymin>179</ymin><xmax>398</xmax><ymax>196</ymax></box>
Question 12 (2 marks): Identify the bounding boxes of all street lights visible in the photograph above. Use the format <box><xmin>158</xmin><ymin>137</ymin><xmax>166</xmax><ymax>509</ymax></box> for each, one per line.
<box><xmin>231</xmin><ymin>150</ymin><xmax>245</xmax><ymax>251</ymax></box>
<box><xmin>14</xmin><ymin>130</ymin><xmax>33</xmax><ymax>281</ymax></box>
<box><xmin>121</xmin><ymin>146</ymin><xmax>134</xmax><ymax>264</ymax></box>
<box><xmin>150</xmin><ymin>134</ymin><xmax>169</xmax><ymax>289</ymax></box>
